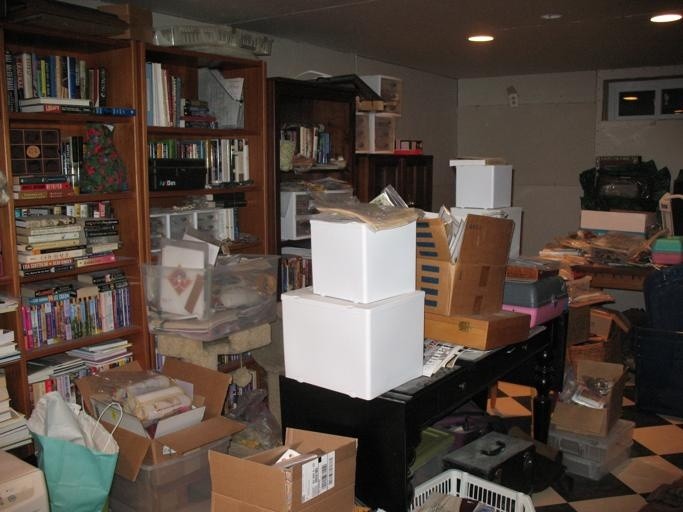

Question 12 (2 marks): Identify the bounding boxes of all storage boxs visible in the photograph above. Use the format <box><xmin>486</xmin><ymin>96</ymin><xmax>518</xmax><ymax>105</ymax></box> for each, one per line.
<box><xmin>409</xmin><ymin>426</ymin><xmax>457</xmax><ymax>489</ymax></box>
<box><xmin>139</xmin><ymin>251</ymin><xmax>283</xmax><ymax>345</ymax></box>
<box><xmin>280</xmin><ymin>188</ymin><xmax>353</xmax><ymax>242</ymax></box>
<box><xmin>71</xmin><ymin>354</ymin><xmax>248</xmax><ymax>512</ymax></box>
<box><xmin>149</xmin><ymin>209</ymin><xmax>227</xmax><ymax>254</ymax></box>
<box><xmin>416</xmin><ymin>214</ymin><xmax>531</xmax><ymax>351</ymax></box>
<box><xmin>280</xmin><ymin>212</ymin><xmax>426</xmax><ymax>402</ymax></box>
<box><xmin>449</xmin><ymin>158</ymin><xmax>523</xmax><ymax>258</ymax></box>
<box><xmin>207</xmin><ymin>426</ymin><xmax>359</xmax><ymax>512</ymax></box>
<box><xmin>440</xmin><ymin>431</ymin><xmax>538</xmax><ymax>497</ymax></box>
<box><xmin>354</xmin><ymin>75</ymin><xmax>402</xmax><ymax>154</ymax></box>
<box><xmin>549</xmin><ymin>358</ymin><xmax>637</xmax><ymax>483</ymax></box>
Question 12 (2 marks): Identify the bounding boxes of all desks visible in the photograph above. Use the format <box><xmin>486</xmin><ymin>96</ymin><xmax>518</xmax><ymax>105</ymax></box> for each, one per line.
<box><xmin>278</xmin><ymin>324</ymin><xmax>550</xmax><ymax>512</ymax></box>
<box><xmin>538</xmin><ymin>232</ymin><xmax>664</xmax><ymax>348</ymax></box>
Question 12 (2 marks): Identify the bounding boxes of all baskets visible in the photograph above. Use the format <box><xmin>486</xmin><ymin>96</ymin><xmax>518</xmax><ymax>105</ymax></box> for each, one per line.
<box><xmin>377</xmin><ymin>467</ymin><xmax>536</xmax><ymax>512</ymax></box>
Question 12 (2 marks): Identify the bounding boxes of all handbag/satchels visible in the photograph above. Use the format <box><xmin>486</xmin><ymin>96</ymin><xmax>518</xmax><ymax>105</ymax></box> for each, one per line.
<box><xmin>24</xmin><ymin>390</ymin><xmax>123</xmax><ymax>512</ymax></box>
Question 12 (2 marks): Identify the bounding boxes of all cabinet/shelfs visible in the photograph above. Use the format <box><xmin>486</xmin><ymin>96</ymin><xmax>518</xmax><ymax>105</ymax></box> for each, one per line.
<box><xmin>267</xmin><ymin>76</ymin><xmax>360</xmax><ymax>303</ymax></box>
<box><xmin>353</xmin><ymin>152</ymin><xmax>434</xmax><ymax>213</ymax></box>
<box><xmin>136</xmin><ymin>41</ymin><xmax>270</xmax><ymax>390</ymax></box>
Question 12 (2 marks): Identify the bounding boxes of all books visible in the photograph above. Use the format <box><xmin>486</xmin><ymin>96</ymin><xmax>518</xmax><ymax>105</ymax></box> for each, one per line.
<box><xmin>146</xmin><ymin>61</ymin><xmax>218</xmax><ymax>129</ymax></box>
<box><xmin>281</xmin><ymin>122</ymin><xmax>331</xmax><ymax>165</ymax></box>
<box><xmin>147</xmin><ymin>137</ymin><xmax>250</xmax><ymax>184</ymax></box>
<box><xmin>4</xmin><ymin>48</ymin><xmax>136</xmax><ymax>117</ymax></box>
<box><xmin>0</xmin><ymin>175</ymin><xmax>134</xmax><ymax>450</ymax></box>
